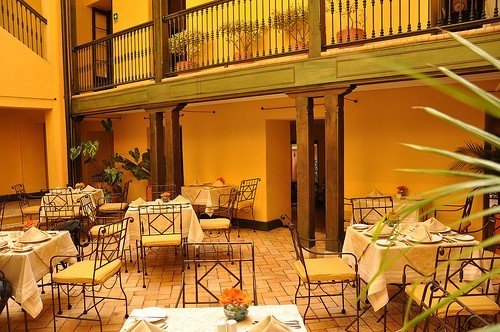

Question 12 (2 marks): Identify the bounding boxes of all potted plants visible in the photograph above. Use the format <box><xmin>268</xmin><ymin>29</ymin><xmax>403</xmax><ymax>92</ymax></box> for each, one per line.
<box><xmin>324</xmin><ymin>0</ymin><xmax>365</xmax><ymax>47</ymax></box>
<box><xmin>168</xmin><ymin>29</ymin><xmax>208</xmax><ymax>70</ymax></box>
<box><xmin>218</xmin><ymin>18</ymin><xmax>264</xmax><ymax>60</ymax></box>
<box><xmin>71</xmin><ymin>141</ymin><xmax>152</xmax><ymax>201</ymax></box>
<box><xmin>273</xmin><ymin>7</ymin><xmax>309</xmax><ymax>51</ymax></box>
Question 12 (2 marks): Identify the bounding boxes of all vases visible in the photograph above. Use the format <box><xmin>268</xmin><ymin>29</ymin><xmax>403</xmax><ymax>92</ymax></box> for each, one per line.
<box><xmin>224</xmin><ymin>306</ymin><xmax>248</xmax><ymax>322</ymax></box>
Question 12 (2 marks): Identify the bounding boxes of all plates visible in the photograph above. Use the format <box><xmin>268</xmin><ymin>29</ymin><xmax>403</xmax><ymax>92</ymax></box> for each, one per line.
<box><xmin>128</xmin><ymin>205</ymin><xmax>138</xmax><ymax>208</ymax></box>
<box><xmin>150</xmin><ymin>207</ymin><xmax>170</xmax><ymax>210</ymax></box>
<box><xmin>352</xmin><ymin>224</ymin><xmax>368</xmax><ymax>228</ymax></box>
<box><xmin>0</xmin><ymin>244</ymin><xmax>10</xmax><ymax>250</ymax></box>
<box><xmin>180</xmin><ymin>203</ymin><xmax>190</xmax><ymax>205</ymax></box>
<box><xmin>428</xmin><ymin>227</ymin><xmax>451</xmax><ymax>233</ymax></box>
<box><xmin>12</xmin><ymin>246</ymin><xmax>33</xmax><ymax>251</ymax></box>
<box><xmin>454</xmin><ymin>234</ymin><xmax>474</xmax><ymax>240</ymax></box>
<box><xmin>212</xmin><ymin>185</ymin><xmax>227</xmax><ymax>187</ymax></box>
<box><xmin>191</xmin><ymin>184</ymin><xmax>205</xmax><ymax>187</ymax></box>
<box><xmin>203</xmin><ymin>187</ymin><xmax>211</xmax><ymax>189</ymax></box>
<box><xmin>18</xmin><ymin>235</ymin><xmax>52</xmax><ymax>242</ymax></box>
<box><xmin>405</xmin><ymin>234</ymin><xmax>442</xmax><ymax>243</ymax></box>
<box><xmin>376</xmin><ymin>240</ymin><xmax>394</xmax><ymax>245</ymax></box>
<box><xmin>363</xmin><ymin>229</ymin><xmax>391</xmax><ymax>238</ymax></box>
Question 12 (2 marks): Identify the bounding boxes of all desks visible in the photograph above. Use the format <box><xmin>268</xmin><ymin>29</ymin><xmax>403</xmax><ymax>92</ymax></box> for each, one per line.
<box><xmin>0</xmin><ymin>230</ymin><xmax>79</xmax><ymax>331</ymax></box>
<box><xmin>180</xmin><ymin>186</ymin><xmax>234</xmax><ymax>217</ymax></box>
<box><xmin>352</xmin><ymin>199</ymin><xmax>432</xmax><ymax>225</ymax></box>
<box><xmin>343</xmin><ymin>225</ymin><xmax>496</xmax><ymax>312</ymax></box>
<box><xmin>41</xmin><ymin>189</ymin><xmax>104</xmax><ymax>222</ymax></box>
<box><xmin>119</xmin><ymin>200</ymin><xmax>204</xmax><ymax>256</ymax></box>
<box><xmin>120</xmin><ymin>304</ymin><xmax>308</xmax><ymax>332</ymax></box>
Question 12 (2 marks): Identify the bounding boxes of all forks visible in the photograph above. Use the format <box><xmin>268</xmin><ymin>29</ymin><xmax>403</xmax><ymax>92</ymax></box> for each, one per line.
<box><xmin>160</xmin><ymin>323</ymin><xmax>168</xmax><ymax>329</ymax></box>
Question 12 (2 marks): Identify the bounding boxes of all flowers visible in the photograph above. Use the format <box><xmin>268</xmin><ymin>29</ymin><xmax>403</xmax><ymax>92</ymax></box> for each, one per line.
<box><xmin>395</xmin><ymin>185</ymin><xmax>408</xmax><ymax>195</ymax></box>
<box><xmin>222</xmin><ymin>288</ymin><xmax>252</xmax><ymax>312</ymax></box>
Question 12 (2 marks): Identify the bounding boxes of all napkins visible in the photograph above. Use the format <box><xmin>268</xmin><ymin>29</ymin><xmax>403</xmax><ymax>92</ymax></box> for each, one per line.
<box><xmin>171</xmin><ymin>194</ymin><xmax>188</xmax><ymax>204</ymax></box>
<box><xmin>410</xmin><ymin>225</ymin><xmax>432</xmax><ymax>241</ymax></box>
<box><xmin>370</xmin><ymin>189</ymin><xmax>384</xmax><ymax>198</ymax></box>
<box><xmin>424</xmin><ymin>216</ymin><xmax>446</xmax><ymax>232</ymax></box>
<box><xmin>247</xmin><ymin>315</ymin><xmax>292</xmax><ymax>332</ymax></box>
<box><xmin>367</xmin><ymin>220</ymin><xmax>392</xmax><ymax>235</ymax></box>
<box><xmin>154</xmin><ymin>198</ymin><xmax>165</xmax><ymax>206</ymax></box>
<box><xmin>20</xmin><ymin>227</ymin><xmax>49</xmax><ymax>241</ymax></box>
<box><xmin>130</xmin><ymin>197</ymin><xmax>147</xmax><ymax>207</ymax></box>
<box><xmin>126</xmin><ymin>320</ymin><xmax>164</xmax><ymax>332</ymax></box>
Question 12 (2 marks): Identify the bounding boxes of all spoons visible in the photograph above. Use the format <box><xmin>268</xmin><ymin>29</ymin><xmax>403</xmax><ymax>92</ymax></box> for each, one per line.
<box><xmin>399</xmin><ymin>239</ymin><xmax>414</xmax><ymax>246</ymax></box>
<box><xmin>441</xmin><ymin>237</ymin><xmax>456</xmax><ymax>243</ymax></box>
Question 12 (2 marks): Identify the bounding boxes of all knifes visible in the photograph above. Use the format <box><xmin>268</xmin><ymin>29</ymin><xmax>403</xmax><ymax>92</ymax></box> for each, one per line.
<box><xmin>252</xmin><ymin>320</ymin><xmax>301</xmax><ymax>328</ymax></box>
<box><xmin>129</xmin><ymin>315</ymin><xmax>167</xmax><ymax>318</ymax></box>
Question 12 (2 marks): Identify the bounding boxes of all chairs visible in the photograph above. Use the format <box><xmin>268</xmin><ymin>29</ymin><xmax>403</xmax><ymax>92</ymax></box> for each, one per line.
<box><xmin>0</xmin><ymin>167</ymin><xmax>500</xmax><ymax>332</ymax></box>
